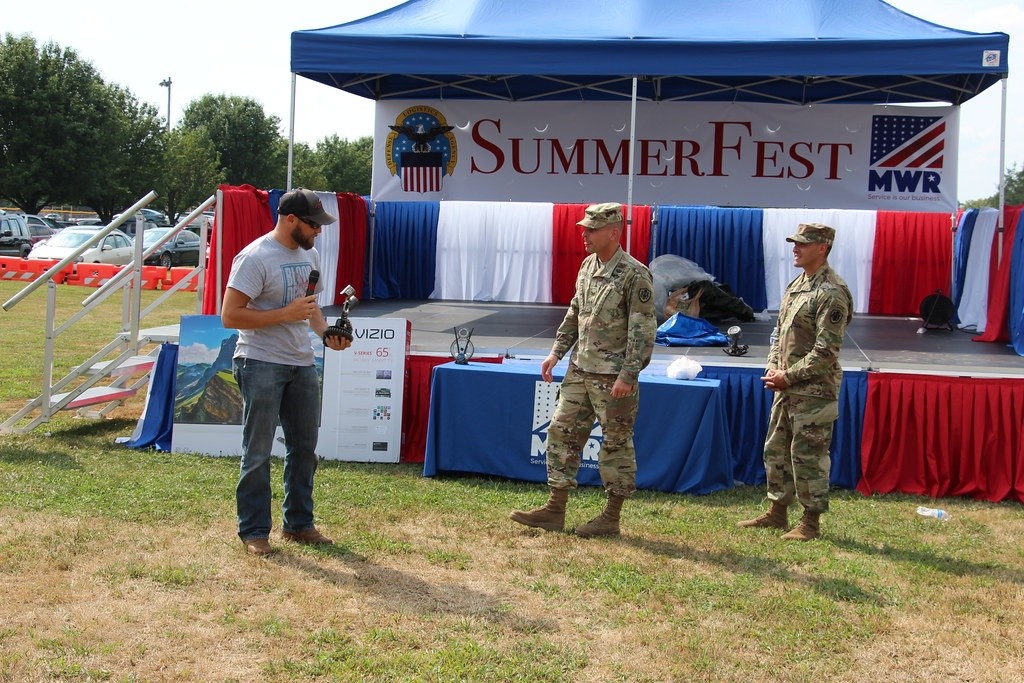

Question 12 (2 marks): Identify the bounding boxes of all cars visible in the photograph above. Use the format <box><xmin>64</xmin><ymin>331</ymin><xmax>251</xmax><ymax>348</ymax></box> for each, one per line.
<box><xmin>16</xmin><ymin>208</ymin><xmax>214</xmax><ymax>271</ymax></box>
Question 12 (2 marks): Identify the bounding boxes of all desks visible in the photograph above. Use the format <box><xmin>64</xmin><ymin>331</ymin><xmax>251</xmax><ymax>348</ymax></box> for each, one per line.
<box><xmin>419</xmin><ymin>359</ymin><xmax>733</xmax><ymax>497</ymax></box>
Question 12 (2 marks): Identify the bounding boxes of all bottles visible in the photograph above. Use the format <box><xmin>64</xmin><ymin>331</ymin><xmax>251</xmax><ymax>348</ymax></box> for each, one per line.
<box><xmin>769</xmin><ymin>326</ymin><xmax>778</xmax><ymax>346</ymax></box>
<box><xmin>917</xmin><ymin>506</ymin><xmax>952</xmax><ymax>519</ymax></box>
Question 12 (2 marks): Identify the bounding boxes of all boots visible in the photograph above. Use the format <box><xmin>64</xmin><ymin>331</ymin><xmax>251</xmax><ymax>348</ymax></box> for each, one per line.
<box><xmin>576</xmin><ymin>494</ymin><xmax>624</xmax><ymax>537</ymax></box>
<box><xmin>738</xmin><ymin>501</ymin><xmax>788</xmax><ymax>529</ymax></box>
<box><xmin>509</xmin><ymin>488</ymin><xmax>569</xmax><ymax>531</ymax></box>
<box><xmin>781</xmin><ymin>511</ymin><xmax>820</xmax><ymax>540</ymax></box>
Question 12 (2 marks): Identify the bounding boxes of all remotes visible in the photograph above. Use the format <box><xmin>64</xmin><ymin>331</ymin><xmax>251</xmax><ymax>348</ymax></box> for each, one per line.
<box><xmin>277</xmin><ymin>437</ymin><xmax>285</xmax><ymax>443</ymax></box>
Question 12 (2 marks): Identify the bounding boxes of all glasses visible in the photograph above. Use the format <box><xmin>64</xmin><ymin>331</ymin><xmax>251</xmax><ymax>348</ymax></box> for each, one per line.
<box><xmin>297</xmin><ymin>217</ymin><xmax>321</xmax><ymax>229</ymax></box>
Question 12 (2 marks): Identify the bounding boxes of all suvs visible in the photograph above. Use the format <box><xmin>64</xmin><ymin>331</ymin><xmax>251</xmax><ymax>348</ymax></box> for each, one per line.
<box><xmin>0</xmin><ymin>209</ymin><xmax>33</xmax><ymax>258</ymax></box>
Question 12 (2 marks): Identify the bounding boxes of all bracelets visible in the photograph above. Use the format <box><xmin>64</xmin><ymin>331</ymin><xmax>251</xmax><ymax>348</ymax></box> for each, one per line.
<box><xmin>783</xmin><ymin>374</ymin><xmax>791</xmax><ymax>386</ymax></box>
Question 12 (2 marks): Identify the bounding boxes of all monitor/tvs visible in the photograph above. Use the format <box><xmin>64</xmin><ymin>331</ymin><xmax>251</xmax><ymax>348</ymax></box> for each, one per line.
<box><xmin>173</xmin><ymin>315</ymin><xmax>327</xmax><ymax>427</ymax></box>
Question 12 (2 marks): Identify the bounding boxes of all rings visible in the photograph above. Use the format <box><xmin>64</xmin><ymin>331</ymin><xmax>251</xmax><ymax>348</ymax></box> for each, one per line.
<box><xmin>622</xmin><ymin>393</ymin><xmax>625</xmax><ymax>395</ymax></box>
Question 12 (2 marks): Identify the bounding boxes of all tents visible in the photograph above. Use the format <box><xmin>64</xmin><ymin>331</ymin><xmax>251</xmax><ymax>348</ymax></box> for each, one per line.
<box><xmin>287</xmin><ymin>1</ymin><xmax>1009</xmax><ymax>300</ymax></box>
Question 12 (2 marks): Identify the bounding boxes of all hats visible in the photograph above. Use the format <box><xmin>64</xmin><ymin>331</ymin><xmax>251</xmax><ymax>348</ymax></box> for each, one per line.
<box><xmin>786</xmin><ymin>224</ymin><xmax>836</xmax><ymax>246</ymax></box>
<box><xmin>277</xmin><ymin>187</ymin><xmax>337</xmax><ymax>225</ymax></box>
<box><xmin>576</xmin><ymin>203</ymin><xmax>622</xmax><ymax>229</ymax></box>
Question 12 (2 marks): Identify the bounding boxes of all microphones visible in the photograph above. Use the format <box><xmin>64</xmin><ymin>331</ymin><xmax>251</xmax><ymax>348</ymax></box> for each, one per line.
<box><xmin>303</xmin><ymin>270</ymin><xmax>320</xmax><ymax>320</ymax></box>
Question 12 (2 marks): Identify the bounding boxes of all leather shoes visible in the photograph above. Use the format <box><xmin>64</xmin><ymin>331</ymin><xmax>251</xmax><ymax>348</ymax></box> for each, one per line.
<box><xmin>281</xmin><ymin>524</ymin><xmax>332</xmax><ymax>547</ymax></box>
<box><xmin>244</xmin><ymin>538</ymin><xmax>272</xmax><ymax>554</ymax></box>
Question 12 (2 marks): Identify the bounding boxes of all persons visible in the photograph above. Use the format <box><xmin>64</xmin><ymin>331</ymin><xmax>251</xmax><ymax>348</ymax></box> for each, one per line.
<box><xmin>222</xmin><ymin>187</ymin><xmax>351</xmax><ymax>555</ymax></box>
<box><xmin>510</xmin><ymin>202</ymin><xmax>657</xmax><ymax>538</ymax></box>
<box><xmin>737</xmin><ymin>223</ymin><xmax>853</xmax><ymax>541</ymax></box>
